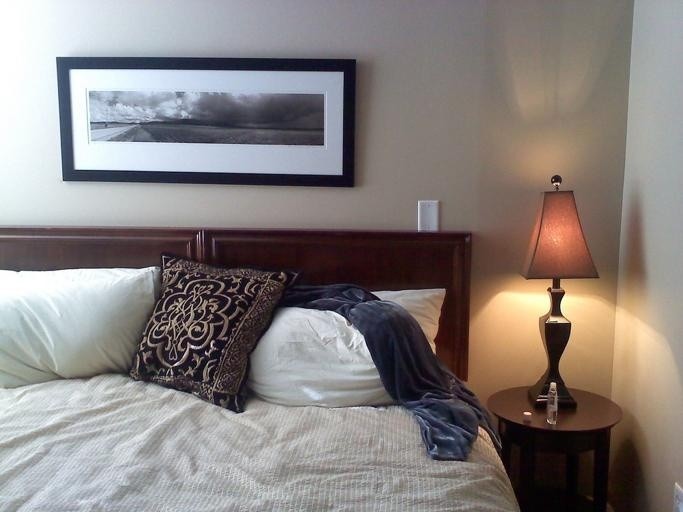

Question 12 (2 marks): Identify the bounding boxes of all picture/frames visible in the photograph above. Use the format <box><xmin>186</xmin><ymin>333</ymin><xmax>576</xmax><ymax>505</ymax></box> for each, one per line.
<box><xmin>57</xmin><ymin>58</ymin><xmax>355</xmax><ymax>187</ymax></box>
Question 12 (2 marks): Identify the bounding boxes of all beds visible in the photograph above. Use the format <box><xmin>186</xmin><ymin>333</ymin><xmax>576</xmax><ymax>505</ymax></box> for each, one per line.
<box><xmin>0</xmin><ymin>226</ymin><xmax>521</xmax><ymax>511</ymax></box>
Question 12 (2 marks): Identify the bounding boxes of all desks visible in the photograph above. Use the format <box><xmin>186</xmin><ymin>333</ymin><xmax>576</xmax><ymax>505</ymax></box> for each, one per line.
<box><xmin>488</xmin><ymin>387</ymin><xmax>623</xmax><ymax>512</ymax></box>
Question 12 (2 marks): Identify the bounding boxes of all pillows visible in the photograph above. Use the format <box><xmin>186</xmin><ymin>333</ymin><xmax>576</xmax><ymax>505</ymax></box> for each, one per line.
<box><xmin>249</xmin><ymin>285</ymin><xmax>447</xmax><ymax>405</ymax></box>
<box><xmin>1</xmin><ymin>264</ymin><xmax>162</xmax><ymax>382</ymax></box>
<box><xmin>128</xmin><ymin>255</ymin><xmax>297</xmax><ymax>414</ymax></box>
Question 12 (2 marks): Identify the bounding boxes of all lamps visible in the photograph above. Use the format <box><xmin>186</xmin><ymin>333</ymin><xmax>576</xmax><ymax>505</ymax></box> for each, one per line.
<box><xmin>520</xmin><ymin>174</ymin><xmax>599</xmax><ymax>411</ymax></box>
